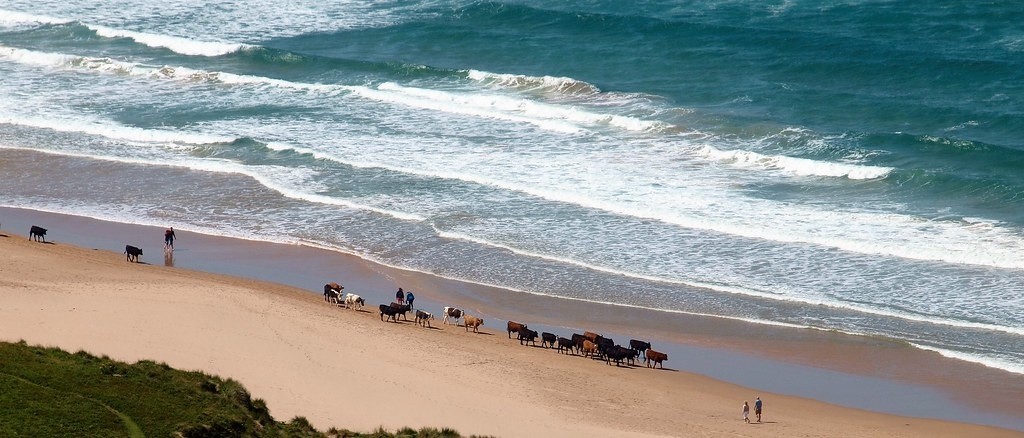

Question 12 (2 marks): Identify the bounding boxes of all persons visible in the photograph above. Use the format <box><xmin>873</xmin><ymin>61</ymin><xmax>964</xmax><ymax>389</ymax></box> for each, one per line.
<box><xmin>395</xmin><ymin>288</ymin><xmax>404</xmax><ymax>304</ymax></box>
<box><xmin>164</xmin><ymin>230</ymin><xmax>171</xmax><ymax>249</ymax></box>
<box><xmin>405</xmin><ymin>290</ymin><xmax>415</xmax><ymax>311</ymax></box>
<box><xmin>169</xmin><ymin>227</ymin><xmax>177</xmax><ymax>250</ymax></box>
<box><xmin>741</xmin><ymin>400</ymin><xmax>750</xmax><ymax>423</ymax></box>
<box><xmin>753</xmin><ymin>396</ymin><xmax>762</xmax><ymax>422</ymax></box>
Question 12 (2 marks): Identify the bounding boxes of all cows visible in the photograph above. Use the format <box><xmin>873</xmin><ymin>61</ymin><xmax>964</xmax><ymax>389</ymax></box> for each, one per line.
<box><xmin>442</xmin><ymin>305</ymin><xmax>485</xmax><ymax>335</ymax></box>
<box><xmin>28</xmin><ymin>225</ymin><xmax>47</xmax><ymax>243</ymax></box>
<box><xmin>507</xmin><ymin>320</ymin><xmax>669</xmax><ymax>369</ymax></box>
<box><xmin>124</xmin><ymin>244</ymin><xmax>143</xmax><ymax>264</ymax></box>
<box><xmin>323</xmin><ymin>281</ymin><xmax>365</xmax><ymax>311</ymax></box>
<box><xmin>379</xmin><ymin>302</ymin><xmax>435</xmax><ymax>329</ymax></box>
